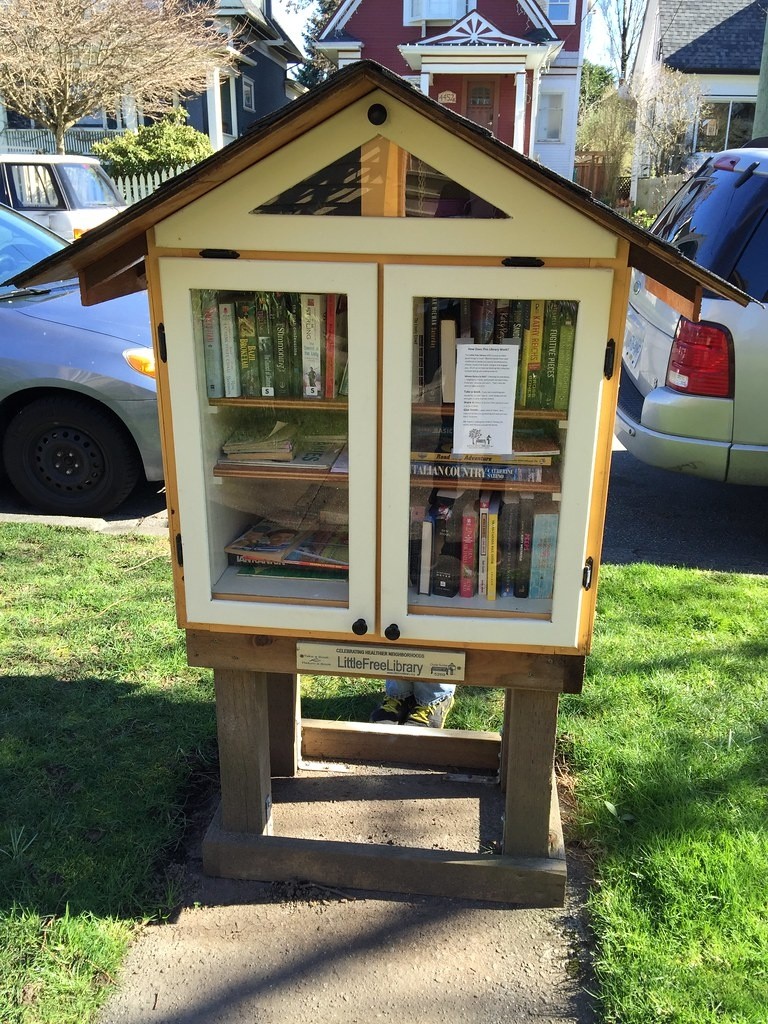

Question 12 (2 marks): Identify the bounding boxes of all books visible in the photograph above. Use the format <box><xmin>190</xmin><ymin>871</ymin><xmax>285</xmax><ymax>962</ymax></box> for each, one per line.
<box><xmin>201</xmin><ymin>289</ymin><xmax>575</xmax><ymax>413</ymax></box>
<box><xmin>224</xmin><ymin>489</ymin><xmax>560</xmax><ymax>599</ymax></box>
<box><xmin>215</xmin><ymin>417</ymin><xmax>561</xmax><ymax>483</ymax></box>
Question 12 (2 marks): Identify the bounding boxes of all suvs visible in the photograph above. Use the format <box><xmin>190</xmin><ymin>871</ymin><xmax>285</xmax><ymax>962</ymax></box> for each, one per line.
<box><xmin>612</xmin><ymin>136</ymin><xmax>768</xmax><ymax>490</ymax></box>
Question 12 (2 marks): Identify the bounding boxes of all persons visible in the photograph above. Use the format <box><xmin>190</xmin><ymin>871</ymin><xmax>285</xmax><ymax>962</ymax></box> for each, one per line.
<box><xmin>368</xmin><ymin>678</ymin><xmax>456</xmax><ymax>730</ymax></box>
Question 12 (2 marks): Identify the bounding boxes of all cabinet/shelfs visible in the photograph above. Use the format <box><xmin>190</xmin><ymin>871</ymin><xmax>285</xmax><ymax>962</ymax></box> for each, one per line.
<box><xmin>150</xmin><ymin>250</ymin><xmax>627</xmax><ymax>654</ymax></box>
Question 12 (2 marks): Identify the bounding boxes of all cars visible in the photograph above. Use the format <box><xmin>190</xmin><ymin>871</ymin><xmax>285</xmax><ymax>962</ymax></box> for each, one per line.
<box><xmin>0</xmin><ymin>153</ymin><xmax>131</xmax><ymax>270</ymax></box>
<box><xmin>1</xmin><ymin>201</ymin><xmax>165</xmax><ymax>519</ymax></box>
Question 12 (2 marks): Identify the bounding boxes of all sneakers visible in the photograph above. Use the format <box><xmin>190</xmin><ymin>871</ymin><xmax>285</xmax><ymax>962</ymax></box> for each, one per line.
<box><xmin>403</xmin><ymin>695</ymin><xmax>455</xmax><ymax>729</ymax></box>
<box><xmin>369</xmin><ymin>691</ymin><xmax>418</xmax><ymax>726</ymax></box>
